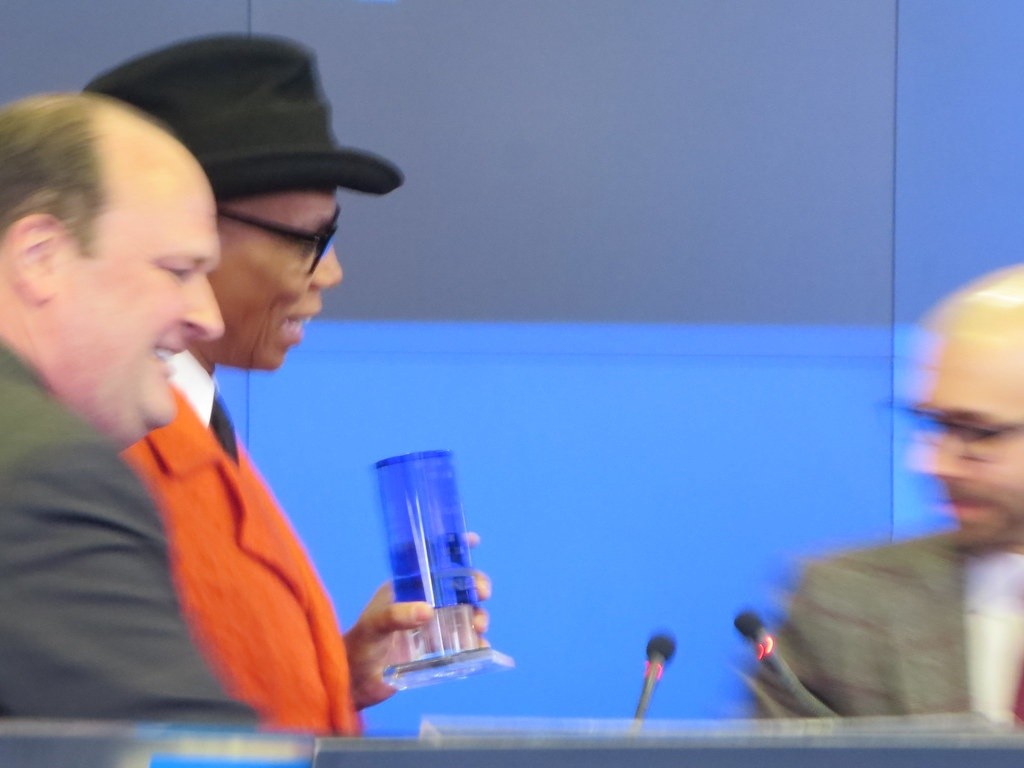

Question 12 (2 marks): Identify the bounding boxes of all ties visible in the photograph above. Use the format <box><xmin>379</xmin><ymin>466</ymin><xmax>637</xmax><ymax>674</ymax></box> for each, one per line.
<box><xmin>212</xmin><ymin>388</ymin><xmax>238</xmax><ymax>465</ymax></box>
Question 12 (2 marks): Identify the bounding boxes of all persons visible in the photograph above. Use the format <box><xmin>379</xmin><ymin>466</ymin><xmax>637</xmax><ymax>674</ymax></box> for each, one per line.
<box><xmin>79</xmin><ymin>30</ymin><xmax>493</xmax><ymax>738</ymax></box>
<box><xmin>754</xmin><ymin>260</ymin><xmax>1023</xmax><ymax>723</ymax></box>
<box><xmin>0</xmin><ymin>91</ymin><xmax>262</xmax><ymax>732</ymax></box>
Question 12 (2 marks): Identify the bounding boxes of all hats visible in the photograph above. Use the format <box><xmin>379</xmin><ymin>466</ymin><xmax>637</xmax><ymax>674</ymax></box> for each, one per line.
<box><xmin>83</xmin><ymin>32</ymin><xmax>404</xmax><ymax>205</ymax></box>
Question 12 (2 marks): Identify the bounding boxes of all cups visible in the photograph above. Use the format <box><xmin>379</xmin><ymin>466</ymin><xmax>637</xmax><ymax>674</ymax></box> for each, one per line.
<box><xmin>370</xmin><ymin>447</ymin><xmax>516</xmax><ymax>694</ymax></box>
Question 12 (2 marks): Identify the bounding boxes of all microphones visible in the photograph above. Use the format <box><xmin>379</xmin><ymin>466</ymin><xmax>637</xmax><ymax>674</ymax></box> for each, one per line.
<box><xmin>635</xmin><ymin>633</ymin><xmax>675</xmax><ymax>720</ymax></box>
<box><xmin>734</xmin><ymin>611</ymin><xmax>838</xmax><ymax>717</ymax></box>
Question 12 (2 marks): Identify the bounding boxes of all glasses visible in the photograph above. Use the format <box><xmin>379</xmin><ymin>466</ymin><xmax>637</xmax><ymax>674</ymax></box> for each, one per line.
<box><xmin>216</xmin><ymin>200</ymin><xmax>341</xmax><ymax>273</ymax></box>
<box><xmin>918</xmin><ymin>415</ymin><xmax>1024</xmax><ymax>445</ymax></box>
